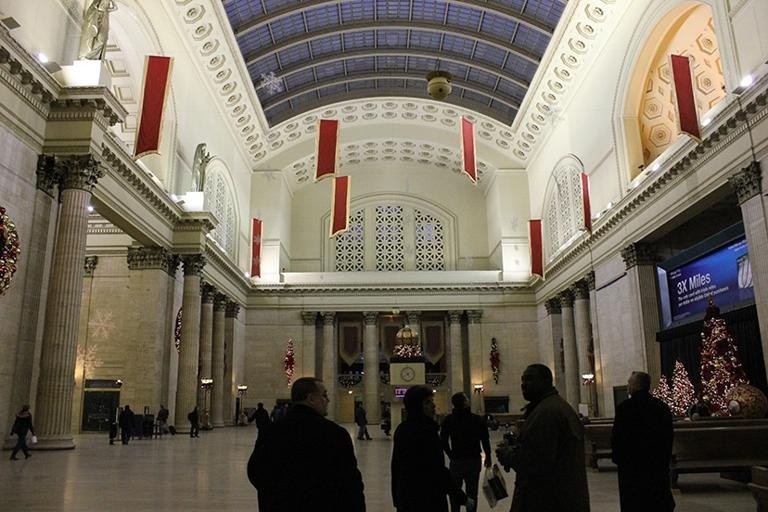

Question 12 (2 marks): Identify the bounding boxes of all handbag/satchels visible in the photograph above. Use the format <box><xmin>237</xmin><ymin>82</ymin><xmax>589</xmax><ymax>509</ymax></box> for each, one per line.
<box><xmin>482</xmin><ymin>469</ymin><xmax>496</xmax><ymax>508</ymax></box>
<box><xmin>485</xmin><ymin>464</ymin><xmax>508</xmax><ymax>501</ymax></box>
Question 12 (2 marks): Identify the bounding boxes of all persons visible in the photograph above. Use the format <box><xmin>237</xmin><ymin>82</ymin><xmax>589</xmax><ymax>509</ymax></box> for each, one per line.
<box><xmin>358</xmin><ymin>401</ymin><xmax>370</xmax><ymax>440</ymax></box>
<box><xmin>687</xmin><ymin>395</ymin><xmax>712</xmax><ymax>418</ymax></box>
<box><xmin>390</xmin><ymin>385</ymin><xmax>476</xmax><ymax>512</ymax></box>
<box><xmin>109</xmin><ymin>418</ymin><xmax>118</xmax><ymax>444</ymax></box>
<box><xmin>187</xmin><ymin>405</ymin><xmax>200</xmax><ymax>437</ymax></box>
<box><xmin>119</xmin><ymin>405</ymin><xmax>134</xmax><ymax>445</ymax></box>
<box><xmin>494</xmin><ymin>363</ymin><xmax>590</xmax><ymax>511</ymax></box>
<box><xmin>246</xmin><ymin>376</ymin><xmax>367</xmax><ymax>510</ymax></box>
<box><xmin>353</xmin><ymin>400</ymin><xmax>366</xmax><ymax>440</ymax></box>
<box><xmin>382</xmin><ymin>403</ymin><xmax>392</xmax><ymax>435</ymax></box>
<box><xmin>8</xmin><ymin>405</ymin><xmax>37</xmax><ymax>460</ymax></box>
<box><xmin>270</xmin><ymin>403</ymin><xmax>290</xmax><ymax>420</ymax></box>
<box><xmin>440</xmin><ymin>391</ymin><xmax>493</xmax><ymax>512</ymax></box>
<box><xmin>245</xmin><ymin>402</ymin><xmax>269</xmax><ymax>432</ymax></box>
<box><xmin>610</xmin><ymin>371</ymin><xmax>676</xmax><ymax>511</ymax></box>
<box><xmin>156</xmin><ymin>404</ymin><xmax>169</xmax><ymax>437</ymax></box>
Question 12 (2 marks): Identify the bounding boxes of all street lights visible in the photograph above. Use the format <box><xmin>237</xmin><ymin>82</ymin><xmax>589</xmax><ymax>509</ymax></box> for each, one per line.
<box><xmin>199</xmin><ymin>378</ymin><xmax>214</xmax><ymax>429</ymax></box>
<box><xmin>581</xmin><ymin>373</ymin><xmax>596</xmax><ymax>418</ymax></box>
<box><xmin>474</xmin><ymin>384</ymin><xmax>485</xmax><ymax>412</ymax></box>
<box><xmin>237</xmin><ymin>384</ymin><xmax>248</xmax><ymax>426</ymax></box>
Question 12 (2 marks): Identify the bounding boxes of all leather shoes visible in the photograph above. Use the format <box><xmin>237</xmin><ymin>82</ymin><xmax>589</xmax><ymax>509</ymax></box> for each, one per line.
<box><xmin>10</xmin><ymin>454</ymin><xmax>20</xmax><ymax>462</ymax></box>
<box><xmin>23</xmin><ymin>454</ymin><xmax>32</xmax><ymax>459</ymax></box>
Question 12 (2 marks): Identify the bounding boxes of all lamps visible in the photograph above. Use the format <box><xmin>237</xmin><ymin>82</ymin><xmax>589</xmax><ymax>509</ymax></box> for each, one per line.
<box><xmin>426</xmin><ymin>59</ymin><xmax>453</xmax><ymax>100</ymax></box>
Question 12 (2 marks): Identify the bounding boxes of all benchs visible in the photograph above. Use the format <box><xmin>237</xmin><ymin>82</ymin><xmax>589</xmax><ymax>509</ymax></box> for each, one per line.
<box><xmin>582</xmin><ymin>417</ymin><xmax>767</xmax><ymax>511</ymax></box>
